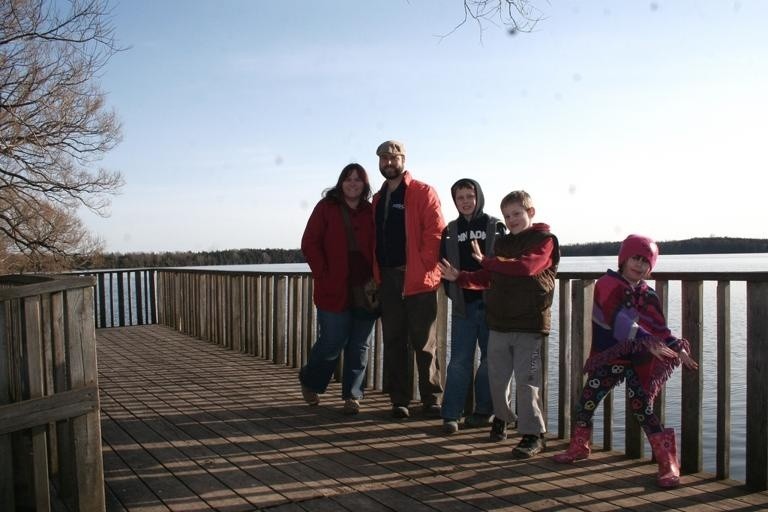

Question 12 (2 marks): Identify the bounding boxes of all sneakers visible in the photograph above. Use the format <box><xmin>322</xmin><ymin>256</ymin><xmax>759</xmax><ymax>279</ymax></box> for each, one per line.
<box><xmin>443</xmin><ymin>418</ymin><xmax>458</xmax><ymax>432</ymax></box>
<box><xmin>466</xmin><ymin>411</ymin><xmax>489</xmax><ymax>425</ymax></box>
<box><xmin>424</xmin><ymin>403</ymin><xmax>441</xmax><ymax>415</ymax></box>
<box><xmin>513</xmin><ymin>434</ymin><xmax>546</xmax><ymax>458</ymax></box>
<box><xmin>392</xmin><ymin>402</ymin><xmax>410</xmax><ymax>416</ymax></box>
<box><xmin>490</xmin><ymin>415</ymin><xmax>507</xmax><ymax>441</ymax></box>
<box><xmin>299</xmin><ymin>376</ymin><xmax>320</xmax><ymax>405</ymax></box>
<box><xmin>342</xmin><ymin>398</ymin><xmax>360</xmax><ymax>413</ymax></box>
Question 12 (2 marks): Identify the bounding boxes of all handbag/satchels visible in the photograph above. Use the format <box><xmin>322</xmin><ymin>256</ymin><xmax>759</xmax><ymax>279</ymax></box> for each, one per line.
<box><xmin>348</xmin><ymin>264</ymin><xmax>383</xmax><ymax>320</ymax></box>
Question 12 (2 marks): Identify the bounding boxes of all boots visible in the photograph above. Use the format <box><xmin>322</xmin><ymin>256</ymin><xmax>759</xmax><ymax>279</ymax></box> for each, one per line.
<box><xmin>648</xmin><ymin>427</ymin><xmax>680</xmax><ymax>485</ymax></box>
<box><xmin>553</xmin><ymin>424</ymin><xmax>593</xmax><ymax>460</ymax></box>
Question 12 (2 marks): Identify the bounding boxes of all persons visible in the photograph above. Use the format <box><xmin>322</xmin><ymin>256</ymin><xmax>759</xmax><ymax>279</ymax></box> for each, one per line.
<box><xmin>300</xmin><ymin>163</ymin><xmax>375</xmax><ymax>415</ymax></box>
<box><xmin>551</xmin><ymin>235</ymin><xmax>699</xmax><ymax>489</ymax></box>
<box><xmin>440</xmin><ymin>179</ymin><xmax>506</xmax><ymax>435</ymax></box>
<box><xmin>369</xmin><ymin>141</ymin><xmax>446</xmax><ymax>418</ymax></box>
<box><xmin>436</xmin><ymin>190</ymin><xmax>560</xmax><ymax>459</ymax></box>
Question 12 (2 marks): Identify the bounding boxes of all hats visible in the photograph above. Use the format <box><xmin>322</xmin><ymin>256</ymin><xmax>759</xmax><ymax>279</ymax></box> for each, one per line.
<box><xmin>376</xmin><ymin>140</ymin><xmax>405</xmax><ymax>158</ymax></box>
<box><xmin>618</xmin><ymin>232</ymin><xmax>658</xmax><ymax>274</ymax></box>
